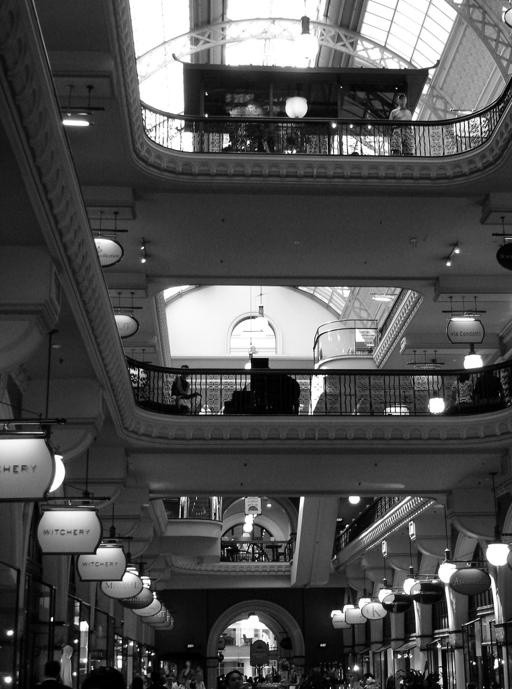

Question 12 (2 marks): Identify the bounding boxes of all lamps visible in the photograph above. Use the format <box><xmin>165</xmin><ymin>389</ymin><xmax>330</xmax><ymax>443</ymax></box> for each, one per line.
<box><xmin>331</xmin><ymin>503</ymin><xmax>458</xmax><ymax>618</ymax></box>
<box><xmin>285</xmin><ymin>83</ymin><xmax>308</xmax><ymax>118</ymax></box>
<box><xmin>463</xmin><ymin>343</ymin><xmax>483</xmax><ymax>370</ymax></box>
<box><xmin>428</xmin><ymin>391</ymin><xmax>446</xmax><ymax>415</ymax></box>
<box><xmin>42</xmin><ymin>328</ymin><xmax>67</xmax><ymax>493</ymax></box>
<box><xmin>484</xmin><ymin>471</ymin><xmax>511</xmax><ymax>567</ymax></box>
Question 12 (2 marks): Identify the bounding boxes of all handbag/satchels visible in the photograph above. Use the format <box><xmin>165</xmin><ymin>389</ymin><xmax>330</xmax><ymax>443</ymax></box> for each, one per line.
<box><xmin>171</xmin><ymin>381</ymin><xmax>188</xmax><ymax>396</ymax></box>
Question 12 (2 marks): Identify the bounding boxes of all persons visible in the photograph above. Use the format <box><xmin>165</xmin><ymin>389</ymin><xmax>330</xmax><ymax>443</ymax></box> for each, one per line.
<box><xmin>39</xmin><ymin>661</ymin><xmax>205</xmax><ymax>689</ymax></box>
<box><xmin>388</xmin><ymin>91</ymin><xmax>416</xmax><ymax>156</ymax></box>
<box><xmin>217</xmin><ymin>670</ymin><xmax>243</xmax><ymax>689</ymax></box>
<box><xmin>171</xmin><ymin>366</ymin><xmax>202</xmax><ymax>416</ymax></box>
<box><xmin>449</xmin><ymin>367</ymin><xmax>506</xmax><ymax>413</ymax></box>
